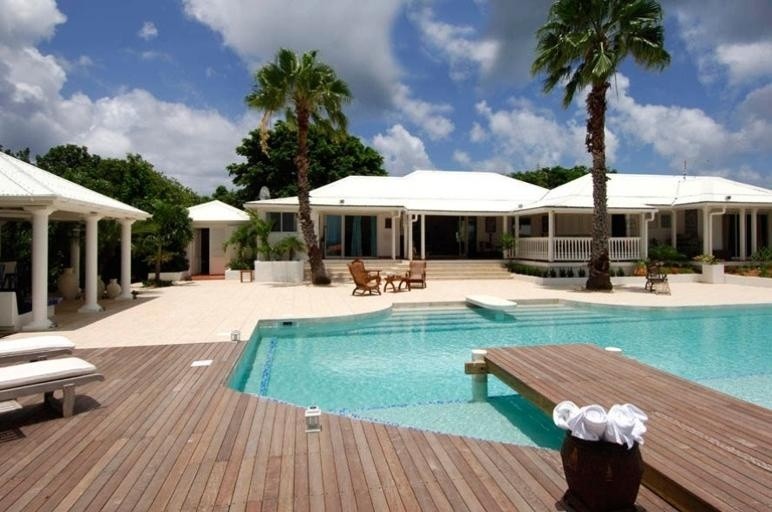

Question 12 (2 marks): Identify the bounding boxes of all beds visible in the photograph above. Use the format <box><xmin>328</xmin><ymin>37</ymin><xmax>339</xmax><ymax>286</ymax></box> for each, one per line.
<box><xmin>0</xmin><ymin>333</ymin><xmax>76</xmax><ymax>365</ymax></box>
<box><xmin>0</xmin><ymin>356</ymin><xmax>105</xmax><ymax>418</ymax></box>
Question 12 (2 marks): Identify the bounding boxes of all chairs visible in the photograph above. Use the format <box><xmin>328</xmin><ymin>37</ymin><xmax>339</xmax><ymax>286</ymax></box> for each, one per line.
<box><xmin>405</xmin><ymin>261</ymin><xmax>427</xmax><ymax>291</ymax></box>
<box><xmin>347</xmin><ymin>259</ymin><xmax>382</xmax><ymax>295</ymax></box>
<box><xmin>645</xmin><ymin>257</ymin><xmax>671</xmax><ymax>294</ymax></box>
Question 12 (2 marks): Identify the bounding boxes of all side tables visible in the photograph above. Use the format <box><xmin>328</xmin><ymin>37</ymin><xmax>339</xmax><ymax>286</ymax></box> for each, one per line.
<box><xmin>384</xmin><ymin>277</ymin><xmax>410</xmax><ymax>293</ymax></box>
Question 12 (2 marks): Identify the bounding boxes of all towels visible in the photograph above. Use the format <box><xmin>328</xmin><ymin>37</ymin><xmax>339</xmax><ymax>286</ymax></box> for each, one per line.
<box><xmin>622</xmin><ymin>403</ymin><xmax>648</xmax><ymax>446</ymax></box>
<box><xmin>572</xmin><ymin>403</ymin><xmax>607</xmax><ymax>441</ymax></box>
<box><xmin>552</xmin><ymin>400</ymin><xmax>579</xmax><ymax>434</ymax></box>
<box><xmin>607</xmin><ymin>403</ymin><xmax>635</xmax><ymax>450</ymax></box>
